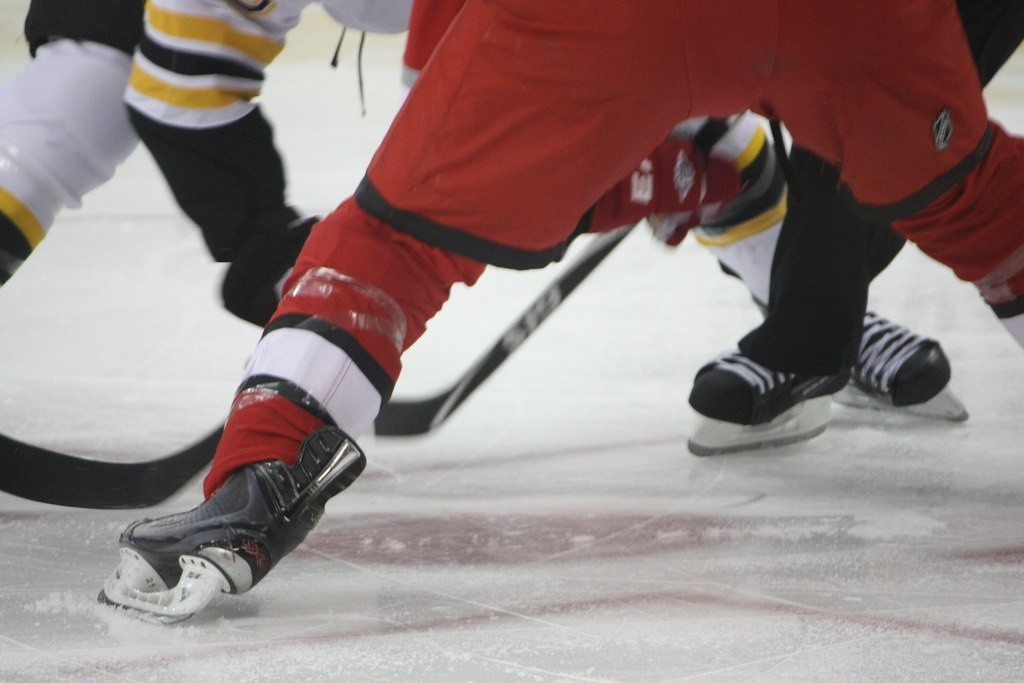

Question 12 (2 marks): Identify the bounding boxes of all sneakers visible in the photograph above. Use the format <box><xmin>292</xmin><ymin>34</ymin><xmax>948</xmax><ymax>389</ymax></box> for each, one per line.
<box><xmin>98</xmin><ymin>427</ymin><xmax>366</xmax><ymax>627</ymax></box>
<box><xmin>688</xmin><ymin>296</ymin><xmax>969</xmax><ymax>457</ymax></box>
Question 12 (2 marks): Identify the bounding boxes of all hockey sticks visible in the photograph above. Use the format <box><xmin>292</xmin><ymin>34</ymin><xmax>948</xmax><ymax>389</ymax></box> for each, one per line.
<box><xmin>0</xmin><ymin>417</ymin><xmax>226</xmax><ymax>512</ymax></box>
<box><xmin>376</xmin><ymin>109</ymin><xmax>748</xmax><ymax>437</ymax></box>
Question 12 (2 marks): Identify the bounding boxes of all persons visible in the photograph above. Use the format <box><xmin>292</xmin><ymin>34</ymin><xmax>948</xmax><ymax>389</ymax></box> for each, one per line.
<box><xmin>0</xmin><ymin>0</ymin><xmax>1024</xmax><ymax>623</ymax></box>
